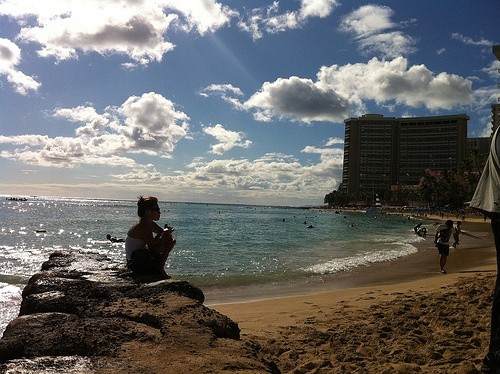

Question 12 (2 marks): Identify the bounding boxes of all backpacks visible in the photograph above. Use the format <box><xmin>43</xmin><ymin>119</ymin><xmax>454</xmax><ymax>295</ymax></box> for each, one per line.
<box><xmin>438</xmin><ymin>228</ymin><xmax>451</xmax><ymax>243</ymax></box>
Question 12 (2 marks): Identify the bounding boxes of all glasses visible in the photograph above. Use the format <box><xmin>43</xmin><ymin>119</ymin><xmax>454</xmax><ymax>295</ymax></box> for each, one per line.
<box><xmin>153</xmin><ymin>207</ymin><xmax>160</xmax><ymax>211</ymax></box>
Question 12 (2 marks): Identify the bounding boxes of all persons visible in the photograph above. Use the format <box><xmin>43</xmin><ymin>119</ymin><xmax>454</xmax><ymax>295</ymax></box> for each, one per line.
<box><xmin>400</xmin><ymin>210</ymin><xmax>466</xmax><ymax>247</ymax></box>
<box><xmin>468</xmin><ymin>41</ymin><xmax>500</xmax><ymax>373</ymax></box>
<box><xmin>434</xmin><ymin>219</ymin><xmax>457</xmax><ymax>274</ymax></box>
<box><xmin>125</xmin><ymin>196</ymin><xmax>176</xmax><ymax>281</ymax></box>
